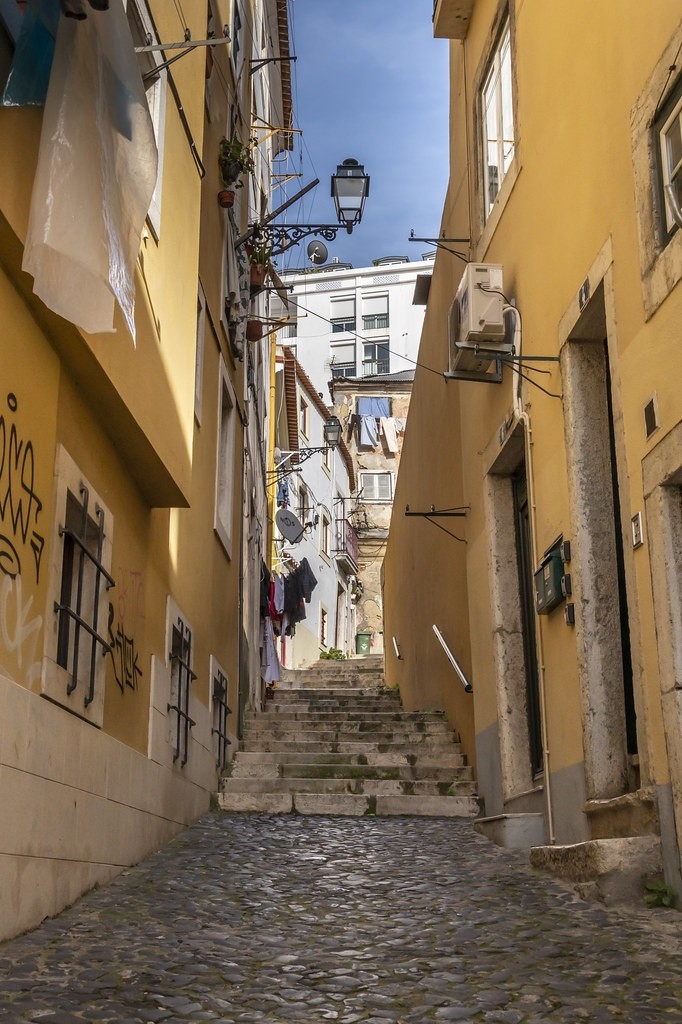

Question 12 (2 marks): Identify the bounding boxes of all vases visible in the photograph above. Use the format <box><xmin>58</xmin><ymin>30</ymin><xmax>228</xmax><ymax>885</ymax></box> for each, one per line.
<box><xmin>247</xmin><ymin>321</ymin><xmax>263</xmax><ymax>341</ymax></box>
<box><xmin>218</xmin><ymin>190</ymin><xmax>235</xmax><ymax>207</ymax></box>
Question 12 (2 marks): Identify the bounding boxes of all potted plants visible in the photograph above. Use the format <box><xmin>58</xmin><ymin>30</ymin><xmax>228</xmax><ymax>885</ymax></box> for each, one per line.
<box><xmin>250</xmin><ymin>248</ymin><xmax>269</xmax><ymax>288</ymax></box>
<box><xmin>218</xmin><ymin>136</ymin><xmax>255</xmax><ymax>189</ymax></box>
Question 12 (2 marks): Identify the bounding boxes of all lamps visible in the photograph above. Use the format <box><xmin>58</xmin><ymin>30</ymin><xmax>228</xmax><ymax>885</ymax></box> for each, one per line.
<box><xmin>249</xmin><ymin>158</ymin><xmax>370</xmax><ymax>258</ymax></box>
<box><xmin>290</xmin><ymin>415</ymin><xmax>343</xmax><ymax>465</ymax></box>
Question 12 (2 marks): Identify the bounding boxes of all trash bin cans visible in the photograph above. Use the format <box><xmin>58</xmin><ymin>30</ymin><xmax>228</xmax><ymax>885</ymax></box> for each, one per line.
<box><xmin>356</xmin><ymin>631</ymin><xmax>372</xmax><ymax>654</ymax></box>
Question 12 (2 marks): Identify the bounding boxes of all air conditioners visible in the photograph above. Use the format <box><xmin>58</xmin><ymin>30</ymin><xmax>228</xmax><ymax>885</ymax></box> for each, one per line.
<box><xmin>447</xmin><ymin>262</ymin><xmax>507</xmax><ymax>373</ymax></box>
